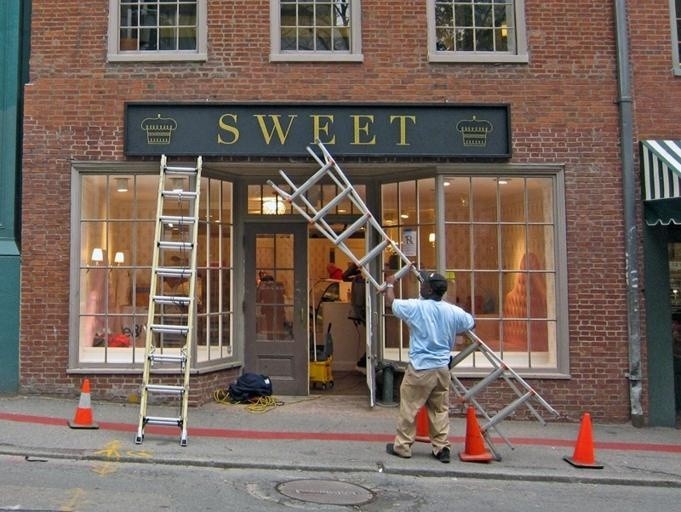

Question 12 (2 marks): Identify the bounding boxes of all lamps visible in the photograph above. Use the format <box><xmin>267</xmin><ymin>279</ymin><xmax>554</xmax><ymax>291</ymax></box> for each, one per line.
<box><xmin>428</xmin><ymin>232</ymin><xmax>435</xmax><ymax>247</ymax></box>
<box><xmin>92</xmin><ymin>248</ymin><xmax>125</xmax><ymax>272</ymax></box>
<box><xmin>116</xmin><ymin>179</ymin><xmax>129</xmax><ymax>191</ymax></box>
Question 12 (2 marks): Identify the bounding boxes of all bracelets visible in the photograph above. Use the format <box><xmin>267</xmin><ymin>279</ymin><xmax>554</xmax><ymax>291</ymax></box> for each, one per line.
<box><xmin>386</xmin><ymin>283</ymin><xmax>395</xmax><ymax>288</ymax></box>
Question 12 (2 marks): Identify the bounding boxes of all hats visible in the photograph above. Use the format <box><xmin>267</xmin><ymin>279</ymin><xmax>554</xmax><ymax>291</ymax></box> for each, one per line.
<box><xmin>420</xmin><ymin>271</ymin><xmax>448</xmax><ymax>296</ymax></box>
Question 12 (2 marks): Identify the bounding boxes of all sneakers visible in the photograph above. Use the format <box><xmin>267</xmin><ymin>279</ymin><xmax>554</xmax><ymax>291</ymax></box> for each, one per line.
<box><xmin>432</xmin><ymin>447</ymin><xmax>451</xmax><ymax>462</ymax></box>
<box><xmin>386</xmin><ymin>443</ymin><xmax>411</xmax><ymax>458</ymax></box>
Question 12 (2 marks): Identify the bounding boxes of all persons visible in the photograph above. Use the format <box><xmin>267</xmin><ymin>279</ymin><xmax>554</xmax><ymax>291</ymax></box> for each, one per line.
<box><xmin>385</xmin><ymin>271</ymin><xmax>478</xmax><ymax>462</ymax></box>
<box><xmin>257</xmin><ymin>275</ymin><xmax>289</xmax><ymax>337</ymax></box>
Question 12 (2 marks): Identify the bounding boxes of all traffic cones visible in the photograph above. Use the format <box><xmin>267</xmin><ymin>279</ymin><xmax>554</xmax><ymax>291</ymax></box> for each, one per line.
<box><xmin>67</xmin><ymin>378</ymin><xmax>100</xmax><ymax>430</ymax></box>
<box><xmin>415</xmin><ymin>404</ymin><xmax>431</xmax><ymax>443</ymax></box>
<box><xmin>459</xmin><ymin>406</ymin><xmax>493</xmax><ymax>462</ymax></box>
<box><xmin>562</xmin><ymin>412</ymin><xmax>604</xmax><ymax>468</ymax></box>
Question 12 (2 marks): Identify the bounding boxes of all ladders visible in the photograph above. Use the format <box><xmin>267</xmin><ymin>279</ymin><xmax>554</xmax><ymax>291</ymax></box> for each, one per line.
<box><xmin>266</xmin><ymin>138</ymin><xmax>560</xmax><ymax>462</ymax></box>
<box><xmin>134</xmin><ymin>155</ymin><xmax>203</xmax><ymax>446</ymax></box>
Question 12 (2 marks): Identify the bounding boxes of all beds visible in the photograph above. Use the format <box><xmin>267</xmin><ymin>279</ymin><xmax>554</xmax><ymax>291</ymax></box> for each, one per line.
<box><xmin>486</xmin><ymin>254</ymin><xmax>546</xmax><ymax>351</ymax></box>
